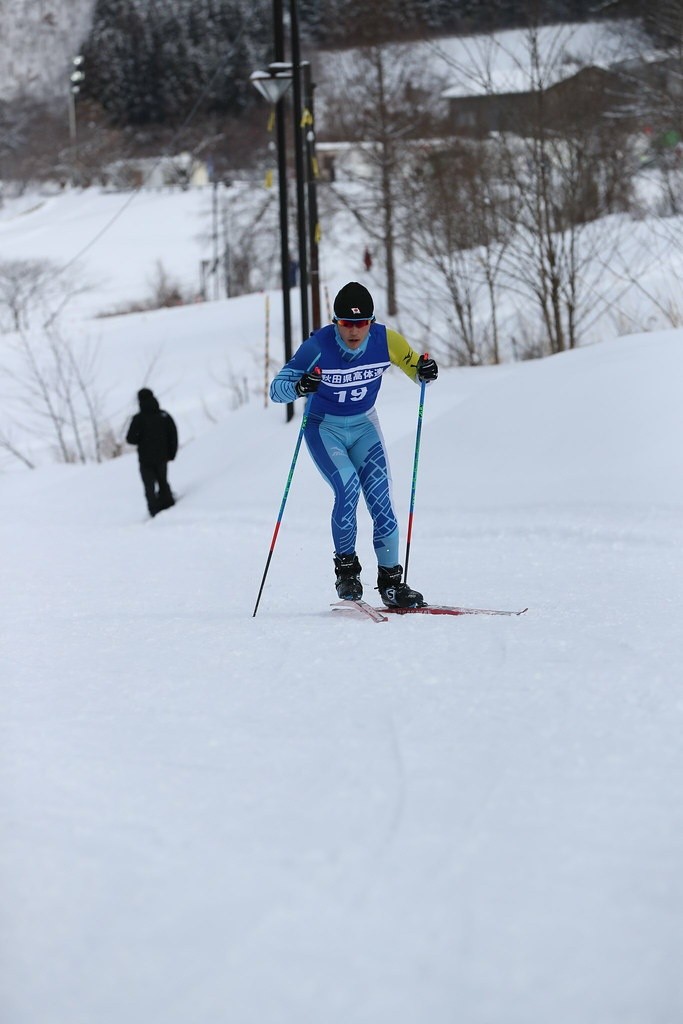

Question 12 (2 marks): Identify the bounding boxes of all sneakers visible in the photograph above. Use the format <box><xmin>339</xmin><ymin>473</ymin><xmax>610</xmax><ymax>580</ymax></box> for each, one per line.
<box><xmin>377</xmin><ymin>565</ymin><xmax>423</xmax><ymax>608</ymax></box>
<box><xmin>334</xmin><ymin>551</ymin><xmax>363</xmax><ymax>600</ymax></box>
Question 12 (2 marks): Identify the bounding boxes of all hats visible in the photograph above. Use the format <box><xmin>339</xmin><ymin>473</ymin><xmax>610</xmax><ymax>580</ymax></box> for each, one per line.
<box><xmin>334</xmin><ymin>282</ymin><xmax>374</xmax><ymax>319</ymax></box>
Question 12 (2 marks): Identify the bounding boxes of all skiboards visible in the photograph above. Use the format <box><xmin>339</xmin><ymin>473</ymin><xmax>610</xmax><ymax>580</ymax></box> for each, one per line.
<box><xmin>330</xmin><ymin>599</ymin><xmax>528</xmax><ymax>624</ymax></box>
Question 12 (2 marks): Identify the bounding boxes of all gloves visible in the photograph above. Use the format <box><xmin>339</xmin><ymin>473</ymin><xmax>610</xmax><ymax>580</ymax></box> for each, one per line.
<box><xmin>299</xmin><ymin>371</ymin><xmax>322</xmax><ymax>393</ymax></box>
<box><xmin>417</xmin><ymin>355</ymin><xmax>438</xmax><ymax>383</ymax></box>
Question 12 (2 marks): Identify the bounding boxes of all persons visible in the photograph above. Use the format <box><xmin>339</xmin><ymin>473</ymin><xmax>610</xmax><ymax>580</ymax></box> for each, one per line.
<box><xmin>124</xmin><ymin>388</ymin><xmax>178</xmax><ymax>514</ymax></box>
<box><xmin>288</xmin><ymin>254</ymin><xmax>298</xmax><ymax>287</ymax></box>
<box><xmin>363</xmin><ymin>249</ymin><xmax>372</xmax><ymax>270</ymax></box>
<box><xmin>269</xmin><ymin>282</ymin><xmax>438</xmax><ymax>609</ymax></box>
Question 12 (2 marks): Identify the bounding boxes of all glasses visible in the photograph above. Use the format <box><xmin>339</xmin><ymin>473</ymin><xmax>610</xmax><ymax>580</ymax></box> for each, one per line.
<box><xmin>337</xmin><ymin>318</ymin><xmax>372</xmax><ymax>329</ymax></box>
